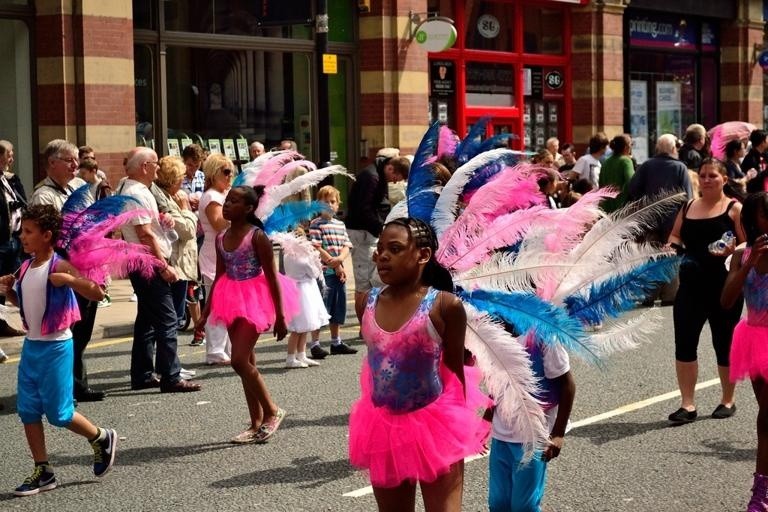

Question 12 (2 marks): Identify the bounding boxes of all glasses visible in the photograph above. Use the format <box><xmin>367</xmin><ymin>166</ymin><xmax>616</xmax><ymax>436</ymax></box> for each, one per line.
<box><xmin>147</xmin><ymin>161</ymin><xmax>160</xmax><ymax>166</ymax></box>
<box><xmin>219</xmin><ymin>169</ymin><xmax>231</xmax><ymax>177</ymax></box>
<box><xmin>52</xmin><ymin>155</ymin><xmax>79</xmax><ymax>164</ymax></box>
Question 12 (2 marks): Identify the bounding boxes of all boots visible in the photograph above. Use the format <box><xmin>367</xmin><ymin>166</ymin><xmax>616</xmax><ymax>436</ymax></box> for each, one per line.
<box><xmin>747</xmin><ymin>473</ymin><xmax>768</xmax><ymax>512</ymax></box>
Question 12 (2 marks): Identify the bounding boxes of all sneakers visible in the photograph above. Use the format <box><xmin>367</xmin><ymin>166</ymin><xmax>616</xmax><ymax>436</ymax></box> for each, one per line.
<box><xmin>330</xmin><ymin>343</ymin><xmax>358</xmax><ymax>355</ymax></box>
<box><xmin>230</xmin><ymin>428</ymin><xmax>258</xmax><ymax>443</ymax></box>
<box><xmin>90</xmin><ymin>427</ymin><xmax>118</xmax><ymax>477</ymax></box>
<box><xmin>13</xmin><ymin>465</ymin><xmax>57</xmax><ymax>499</ymax></box>
<box><xmin>286</xmin><ymin>360</ymin><xmax>309</xmax><ymax>368</ymax></box>
<box><xmin>711</xmin><ymin>403</ymin><xmax>737</xmax><ymax>420</ymax></box>
<box><xmin>96</xmin><ymin>293</ymin><xmax>114</xmax><ymax>308</ymax></box>
<box><xmin>296</xmin><ymin>358</ymin><xmax>320</xmax><ymax>366</ymax></box>
<box><xmin>666</xmin><ymin>407</ymin><xmax>698</xmax><ymax>424</ymax></box>
<box><xmin>176</xmin><ymin>368</ymin><xmax>198</xmax><ymax>379</ymax></box>
<box><xmin>127</xmin><ymin>293</ymin><xmax>138</xmax><ymax>304</ymax></box>
<box><xmin>309</xmin><ymin>345</ymin><xmax>329</xmax><ymax>359</ymax></box>
<box><xmin>190</xmin><ymin>332</ymin><xmax>206</xmax><ymax>346</ymax></box>
<box><xmin>254</xmin><ymin>406</ymin><xmax>287</xmax><ymax>444</ymax></box>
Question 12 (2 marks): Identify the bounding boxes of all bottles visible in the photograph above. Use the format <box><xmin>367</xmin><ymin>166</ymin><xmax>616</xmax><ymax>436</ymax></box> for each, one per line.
<box><xmin>723</xmin><ymin>231</ymin><xmax>735</xmax><ymax>246</ymax></box>
<box><xmin>159</xmin><ymin>213</ymin><xmax>179</xmax><ymax>243</ymax></box>
<box><xmin>707</xmin><ymin>240</ymin><xmax>728</xmax><ymax>256</ymax></box>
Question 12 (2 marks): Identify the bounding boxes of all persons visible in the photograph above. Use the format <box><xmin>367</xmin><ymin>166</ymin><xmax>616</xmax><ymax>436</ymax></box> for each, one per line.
<box><xmin>346</xmin><ymin>218</ymin><xmax>490</xmax><ymax>512</ymax></box>
<box><xmin>477</xmin><ymin>284</ymin><xmax>574</xmax><ymax>510</ymax></box>
<box><xmin>718</xmin><ymin>193</ymin><xmax>768</xmax><ymax>510</ymax></box>
<box><xmin>659</xmin><ymin>158</ymin><xmax>748</xmax><ymax>422</ymax></box>
<box><xmin>346</xmin><ymin>124</ymin><xmax>766</xmax><ymax>340</ymax></box>
<box><xmin>2</xmin><ymin>202</ymin><xmax>116</xmax><ymax>496</ymax></box>
<box><xmin>195</xmin><ymin>185</ymin><xmax>289</xmax><ymax>445</ymax></box>
<box><xmin>0</xmin><ymin>138</ymin><xmax>358</xmax><ymax>402</ymax></box>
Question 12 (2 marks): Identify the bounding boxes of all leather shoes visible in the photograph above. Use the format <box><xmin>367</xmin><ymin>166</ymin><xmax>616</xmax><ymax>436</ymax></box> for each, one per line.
<box><xmin>74</xmin><ymin>387</ymin><xmax>106</xmax><ymax>402</ymax></box>
<box><xmin>159</xmin><ymin>378</ymin><xmax>202</xmax><ymax>393</ymax></box>
<box><xmin>129</xmin><ymin>375</ymin><xmax>160</xmax><ymax>390</ymax></box>
